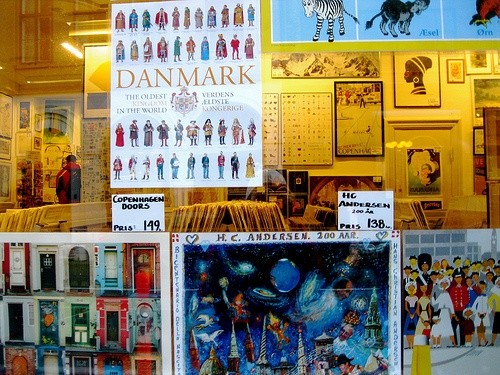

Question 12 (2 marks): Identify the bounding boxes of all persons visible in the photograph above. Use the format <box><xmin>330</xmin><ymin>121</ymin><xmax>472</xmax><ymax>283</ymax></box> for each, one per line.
<box><xmin>56</xmin><ymin>155</ymin><xmax>81</xmax><ymax>203</ymax></box>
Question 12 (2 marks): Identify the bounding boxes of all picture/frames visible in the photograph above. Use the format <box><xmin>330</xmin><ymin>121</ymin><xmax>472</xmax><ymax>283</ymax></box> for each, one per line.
<box><xmin>392</xmin><ymin>51</ymin><xmax>441</xmax><ymax>107</ymax></box>
<box><xmin>271</xmin><ymin>52</ymin><xmax>381</xmax><ymax>78</ymax></box>
<box><xmin>446</xmin><ymin>51</ymin><xmax>500</xmax><ymax>84</ymax></box>
<box><xmin>0</xmin><ymin>92</ymin><xmax>43</xmax><ymax>201</ymax></box>
<box><xmin>473</xmin><ymin>126</ymin><xmax>484</xmax><ymax>156</ymax></box>
<box><xmin>333</xmin><ymin>81</ymin><xmax>385</xmax><ymax>156</ymax></box>
<box><xmin>43</xmin><ymin>97</ymin><xmax>75</xmax><ymax>145</ymax></box>
<box><xmin>406</xmin><ymin>147</ymin><xmax>444</xmax><ymax>199</ymax></box>
<box><xmin>83</xmin><ymin>44</ymin><xmax>110</xmax><ymax>118</ymax></box>
